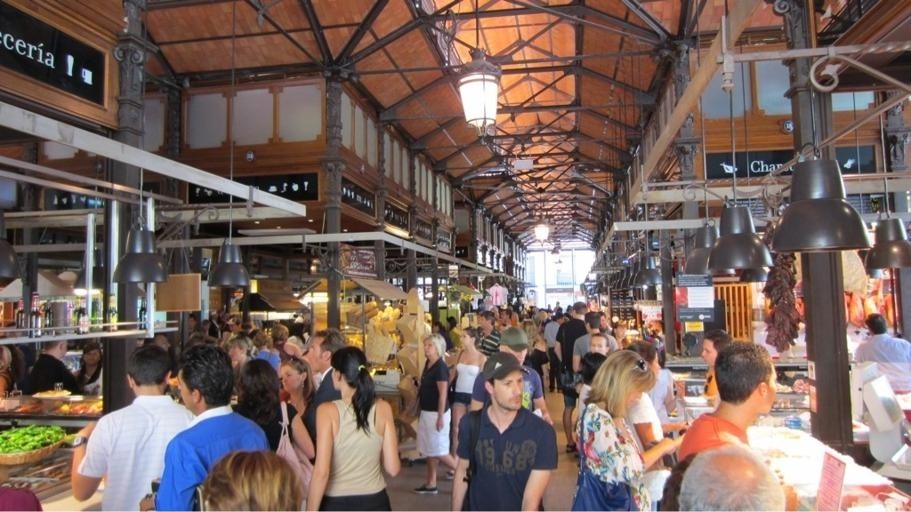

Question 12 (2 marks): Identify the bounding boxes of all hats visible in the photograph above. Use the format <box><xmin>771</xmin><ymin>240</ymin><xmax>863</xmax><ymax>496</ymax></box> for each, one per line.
<box><xmin>482</xmin><ymin>351</ymin><xmax>531</xmax><ymax>380</ymax></box>
<box><xmin>498</xmin><ymin>326</ymin><xmax>530</xmax><ymax>352</ymax></box>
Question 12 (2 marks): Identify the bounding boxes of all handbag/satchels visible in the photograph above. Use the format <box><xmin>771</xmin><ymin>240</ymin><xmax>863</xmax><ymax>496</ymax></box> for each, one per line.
<box><xmin>274</xmin><ymin>400</ymin><xmax>314</xmax><ymax>502</ymax></box>
<box><xmin>569</xmin><ymin>406</ymin><xmax>643</xmax><ymax>511</ymax></box>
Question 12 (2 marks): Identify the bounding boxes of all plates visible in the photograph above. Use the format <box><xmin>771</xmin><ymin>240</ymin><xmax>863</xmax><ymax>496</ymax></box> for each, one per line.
<box><xmin>32</xmin><ymin>388</ymin><xmax>73</xmax><ymax>398</ymax></box>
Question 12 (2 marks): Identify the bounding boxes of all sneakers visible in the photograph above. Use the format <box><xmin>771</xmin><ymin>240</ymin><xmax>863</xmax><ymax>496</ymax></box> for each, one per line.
<box><xmin>411</xmin><ymin>483</ymin><xmax>439</xmax><ymax>495</ymax></box>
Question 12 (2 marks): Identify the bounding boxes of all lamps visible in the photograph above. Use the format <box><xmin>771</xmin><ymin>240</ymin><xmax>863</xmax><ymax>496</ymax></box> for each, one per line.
<box><xmin>683</xmin><ymin>96</ymin><xmax>738</xmax><ymax>278</ymax></box>
<box><xmin>205</xmin><ymin>0</ymin><xmax>250</xmax><ymax>287</ymax></box>
<box><xmin>111</xmin><ymin>0</ymin><xmax>170</xmax><ymax>283</ymax></box>
<box><xmin>0</xmin><ymin>237</ymin><xmax>22</xmax><ymax>279</ymax></box>
<box><xmin>863</xmin><ymin>90</ymin><xmax>911</xmax><ymax>269</ymax></box>
<box><xmin>534</xmin><ymin>220</ymin><xmax>549</xmax><ymax>245</ymax></box>
<box><xmin>457</xmin><ymin>0</ymin><xmax>503</xmax><ymax>137</ymax></box>
<box><xmin>73</xmin><ymin>156</ymin><xmax>104</xmax><ymax>289</ymax></box>
<box><xmin>705</xmin><ymin>60</ymin><xmax>774</xmax><ymax>283</ymax></box>
<box><xmin>770</xmin><ymin>58</ymin><xmax>874</xmax><ymax>253</ymax></box>
<box><xmin>584</xmin><ymin>189</ymin><xmax>664</xmax><ymax>295</ymax></box>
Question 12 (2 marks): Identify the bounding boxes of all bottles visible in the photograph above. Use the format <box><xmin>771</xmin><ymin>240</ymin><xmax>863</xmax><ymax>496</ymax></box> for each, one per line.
<box><xmin>0</xmin><ymin>295</ymin><xmax>153</xmax><ymax>336</ymax></box>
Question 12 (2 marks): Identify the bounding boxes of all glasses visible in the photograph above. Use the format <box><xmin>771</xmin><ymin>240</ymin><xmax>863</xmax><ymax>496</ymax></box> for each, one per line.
<box><xmin>631</xmin><ymin>359</ymin><xmax>647</xmax><ymax>373</ymax></box>
<box><xmin>703</xmin><ymin>374</ymin><xmax>712</xmax><ymax>394</ymax></box>
